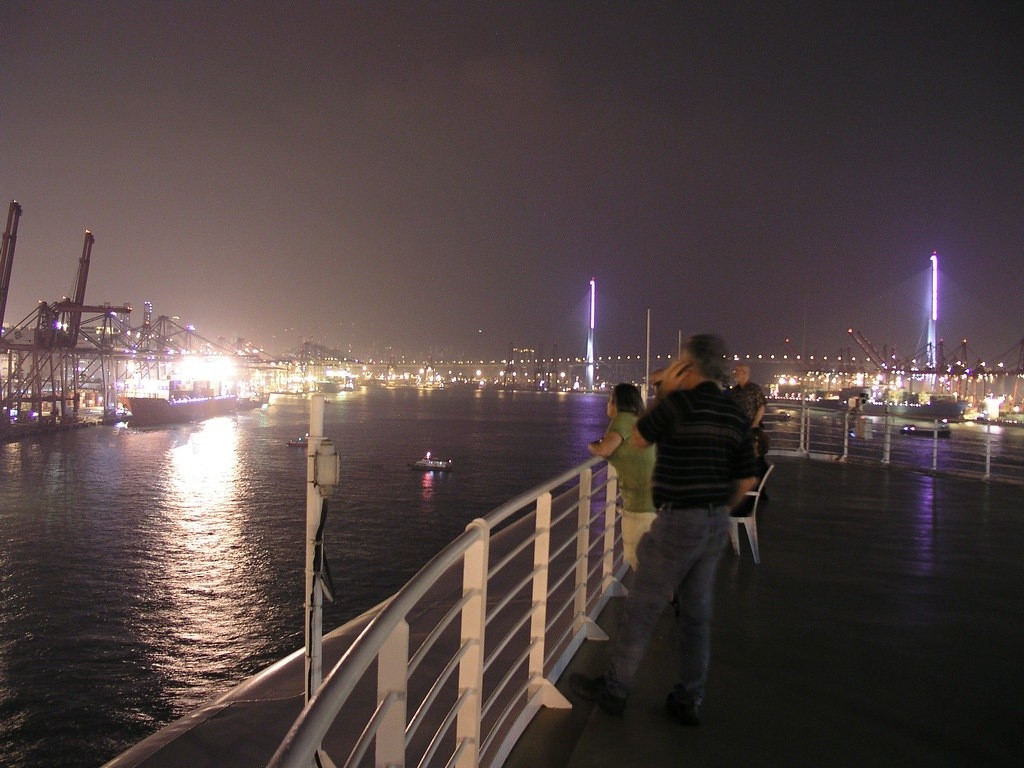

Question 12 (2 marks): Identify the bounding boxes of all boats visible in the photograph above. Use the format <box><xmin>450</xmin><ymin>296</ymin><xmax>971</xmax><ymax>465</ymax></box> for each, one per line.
<box><xmin>813</xmin><ymin>378</ymin><xmax>969</xmax><ymax>424</ymax></box>
<box><xmin>407</xmin><ymin>452</ymin><xmax>452</xmax><ymax>472</ymax></box>
<box><xmin>265</xmin><ymin>340</ymin><xmax>622</xmax><ymax>397</ymax></box>
<box><xmin>900</xmin><ymin>423</ymin><xmax>952</xmax><ymax>437</ymax></box>
<box><xmin>116</xmin><ymin>378</ymin><xmax>271</xmax><ymax>423</ymax></box>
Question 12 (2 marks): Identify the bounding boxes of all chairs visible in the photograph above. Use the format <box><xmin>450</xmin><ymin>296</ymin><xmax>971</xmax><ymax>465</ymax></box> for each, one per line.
<box><xmin>728</xmin><ymin>463</ymin><xmax>775</xmax><ymax>564</ymax></box>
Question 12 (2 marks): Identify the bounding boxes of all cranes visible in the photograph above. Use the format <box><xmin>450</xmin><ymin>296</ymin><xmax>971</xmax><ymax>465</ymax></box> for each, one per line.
<box><xmin>847</xmin><ymin>328</ymin><xmax>1024</xmax><ymax>375</ymax></box>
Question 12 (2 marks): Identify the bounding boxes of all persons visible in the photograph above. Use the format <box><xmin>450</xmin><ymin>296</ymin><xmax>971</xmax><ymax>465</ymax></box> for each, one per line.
<box><xmin>571</xmin><ymin>332</ymin><xmax>759</xmax><ymax>725</ymax></box>
<box><xmin>729</xmin><ymin>428</ymin><xmax>770</xmax><ymax>517</ymax></box>
<box><xmin>728</xmin><ymin>359</ymin><xmax>767</xmax><ymax>429</ymax></box>
<box><xmin>588</xmin><ymin>383</ymin><xmax>656</xmax><ymax>571</ymax></box>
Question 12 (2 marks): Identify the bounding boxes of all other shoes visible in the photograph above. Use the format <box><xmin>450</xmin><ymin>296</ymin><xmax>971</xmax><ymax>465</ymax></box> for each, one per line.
<box><xmin>568</xmin><ymin>674</ymin><xmax>628</xmax><ymax>717</ymax></box>
<box><xmin>663</xmin><ymin>685</ymin><xmax>703</xmax><ymax>727</ymax></box>
<box><xmin>670</xmin><ymin>589</ymin><xmax>681</xmax><ymax>618</ymax></box>
